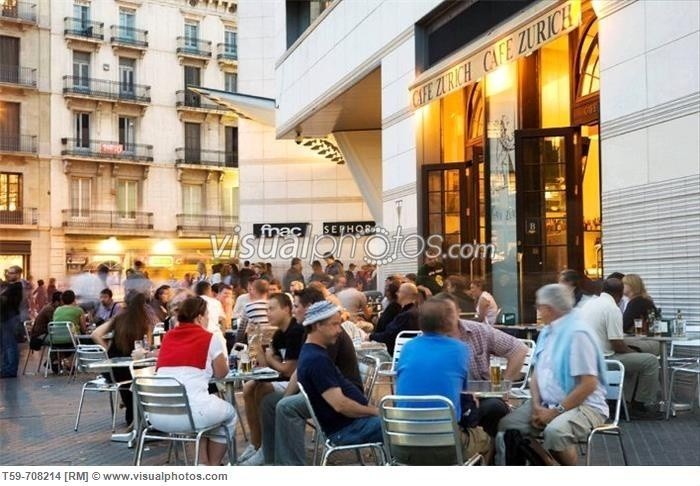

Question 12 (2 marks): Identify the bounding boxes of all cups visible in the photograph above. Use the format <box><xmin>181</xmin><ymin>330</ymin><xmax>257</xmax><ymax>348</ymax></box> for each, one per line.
<box><xmin>489</xmin><ymin>356</ymin><xmax>501</xmax><ymax>387</ymax></box>
<box><xmin>229</xmin><ymin>350</ymin><xmax>257</xmax><ymax>374</ymax></box>
<box><xmin>134</xmin><ymin>334</ymin><xmax>160</xmax><ymax>351</ymax></box>
<box><xmin>504</xmin><ymin>375</ymin><xmax>512</xmax><ymax>389</ymax></box>
<box><xmin>231</xmin><ymin>318</ymin><xmax>237</xmax><ymax>329</ymax></box>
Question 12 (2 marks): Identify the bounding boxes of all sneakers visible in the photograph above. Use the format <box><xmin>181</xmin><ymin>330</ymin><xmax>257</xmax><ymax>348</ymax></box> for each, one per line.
<box><xmin>631</xmin><ymin>407</ymin><xmax>665</xmax><ymax>423</ymax></box>
<box><xmin>236</xmin><ymin>444</ymin><xmax>265</xmax><ymax>465</ymax></box>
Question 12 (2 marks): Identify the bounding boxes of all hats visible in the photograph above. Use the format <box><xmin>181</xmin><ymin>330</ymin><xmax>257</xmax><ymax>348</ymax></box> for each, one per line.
<box><xmin>302</xmin><ymin>299</ymin><xmax>342</xmax><ymax>328</ymax></box>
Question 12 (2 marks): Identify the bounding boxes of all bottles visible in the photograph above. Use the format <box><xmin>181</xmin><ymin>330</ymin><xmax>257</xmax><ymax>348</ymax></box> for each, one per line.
<box><xmin>86</xmin><ymin>323</ymin><xmax>96</xmax><ymax>335</ymax></box>
<box><xmin>367</xmin><ymin>295</ymin><xmax>382</xmax><ymax>312</ymax></box>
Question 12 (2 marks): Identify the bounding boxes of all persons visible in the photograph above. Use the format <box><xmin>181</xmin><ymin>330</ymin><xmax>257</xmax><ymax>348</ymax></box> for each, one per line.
<box><xmin>0</xmin><ymin>253</ymin><xmax>665</xmax><ymax>466</ymax></box>
